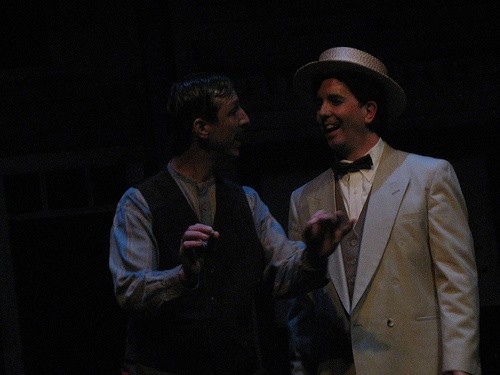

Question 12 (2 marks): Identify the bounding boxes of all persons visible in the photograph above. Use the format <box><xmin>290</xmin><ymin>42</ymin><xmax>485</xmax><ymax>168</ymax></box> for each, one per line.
<box><xmin>274</xmin><ymin>46</ymin><xmax>484</xmax><ymax>375</ymax></box>
<box><xmin>106</xmin><ymin>71</ymin><xmax>363</xmax><ymax>375</ymax></box>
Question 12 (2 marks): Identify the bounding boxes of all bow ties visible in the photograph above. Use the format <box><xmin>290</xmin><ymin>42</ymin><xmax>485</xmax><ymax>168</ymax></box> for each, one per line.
<box><xmin>336</xmin><ymin>152</ymin><xmax>373</xmax><ymax>176</ymax></box>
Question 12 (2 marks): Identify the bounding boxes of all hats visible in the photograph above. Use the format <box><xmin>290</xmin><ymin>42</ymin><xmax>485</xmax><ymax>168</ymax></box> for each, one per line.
<box><xmin>294</xmin><ymin>47</ymin><xmax>406</xmax><ymax>124</ymax></box>
